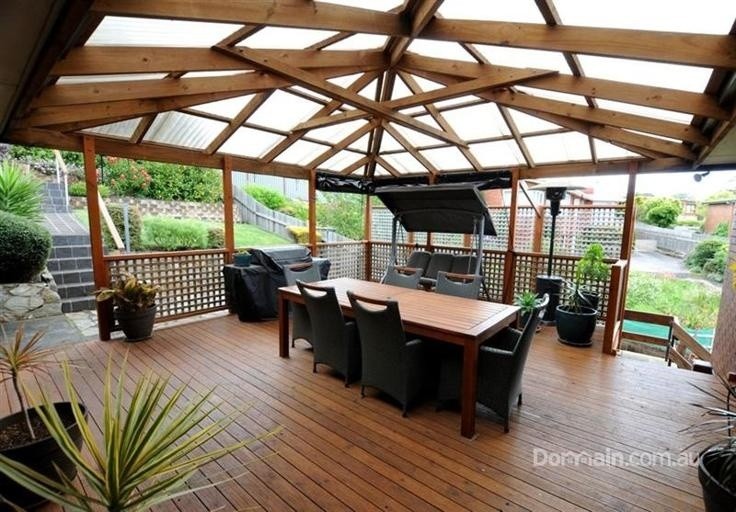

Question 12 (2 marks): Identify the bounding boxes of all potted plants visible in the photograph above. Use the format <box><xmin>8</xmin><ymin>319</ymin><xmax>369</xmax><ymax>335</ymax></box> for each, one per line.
<box><xmin>575</xmin><ymin>242</ymin><xmax>612</xmax><ymax>311</ymax></box>
<box><xmin>0</xmin><ymin>322</ymin><xmax>88</xmax><ymax>510</ymax></box>
<box><xmin>90</xmin><ymin>271</ymin><xmax>159</xmax><ymax>342</ymax></box>
<box><xmin>551</xmin><ymin>271</ymin><xmax>602</xmax><ymax>347</ymax></box>
<box><xmin>674</xmin><ymin>368</ymin><xmax>736</xmax><ymax>511</ymax></box>
<box><xmin>513</xmin><ymin>289</ymin><xmax>542</xmax><ymax>332</ymax></box>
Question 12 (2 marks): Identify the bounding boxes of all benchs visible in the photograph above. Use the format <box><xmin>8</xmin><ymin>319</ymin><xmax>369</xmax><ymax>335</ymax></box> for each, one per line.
<box><xmin>394</xmin><ymin>251</ymin><xmax>478</xmax><ymax>286</ymax></box>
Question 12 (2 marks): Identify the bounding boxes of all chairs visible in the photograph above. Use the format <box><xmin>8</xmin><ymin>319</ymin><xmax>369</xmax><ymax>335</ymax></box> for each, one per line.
<box><xmin>283</xmin><ymin>261</ymin><xmax>321</xmax><ymax>350</ymax></box>
<box><xmin>434</xmin><ymin>270</ymin><xmax>484</xmax><ymax>299</ymax></box>
<box><xmin>294</xmin><ymin>276</ymin><xmax>359</xmax><ymax>387</ymax></box>
<box><xmin>342</xmin><ymin>289</ymin><xmax>433</xmax><ymax>418</ymax></box>
<box><xmin>401</xmin><ymin>248</ymin><xmax>431</xmax><ymax>284</ymax></box>
<box><xmin>448</xmin><ymin>254</ymin><xmax>479</xmax><ymax>284</ymax></box>
<box><xmin>430</xmin><ymin>291</ymin><xmax>549</xmax><ymax>434</ymax></box>
<box><xmin>380</xmin><ymin>265</ymin><xmax>424</xmax><ymax>289</ymax></box>
<box><xmin>421</xmin><ymin>252</ymin><xmax>456</xmax><ymax>289</ymax></box>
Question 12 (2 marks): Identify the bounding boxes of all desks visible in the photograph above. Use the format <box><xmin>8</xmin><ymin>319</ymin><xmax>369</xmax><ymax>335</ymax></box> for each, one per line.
<box><xmin>221</xmin><ymin>244</ymin><xmax>332</xmax><ymax>323</ymax></box>
<box><xmin>277</xmin><ymin>276</ymin><xmax>524</xmax><ymax>442</ymax></box>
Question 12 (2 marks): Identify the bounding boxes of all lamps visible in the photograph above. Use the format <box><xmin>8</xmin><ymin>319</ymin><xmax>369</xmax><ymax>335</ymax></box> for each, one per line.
<box><xmin>527</xmin><ymin>181</ymin><xmax>589</xmax><ymax>325</ymax></box>
